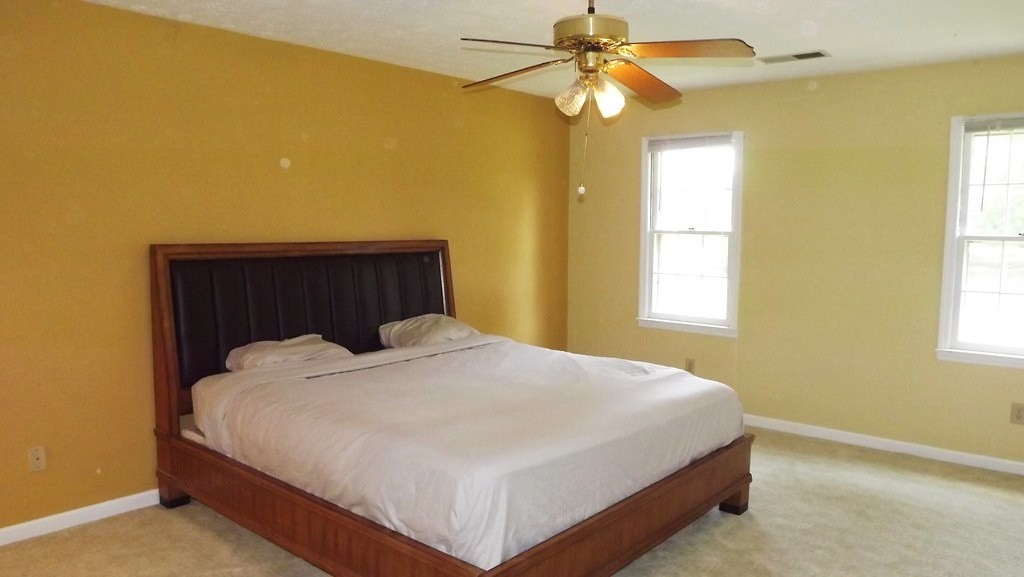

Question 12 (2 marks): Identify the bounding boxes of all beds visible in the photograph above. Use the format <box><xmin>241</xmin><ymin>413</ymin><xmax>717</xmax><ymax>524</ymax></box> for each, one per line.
<box><xmin>150</xmin><ymin>239</ymin><xmax>754</xmax><ymax>577</ymax></box>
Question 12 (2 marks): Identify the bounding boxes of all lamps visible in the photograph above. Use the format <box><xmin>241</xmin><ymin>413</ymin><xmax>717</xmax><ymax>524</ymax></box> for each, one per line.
<box><xmin>554</xmin><ymin>69</ymin><xmax>625</xmax><ymax>118</ymax></box>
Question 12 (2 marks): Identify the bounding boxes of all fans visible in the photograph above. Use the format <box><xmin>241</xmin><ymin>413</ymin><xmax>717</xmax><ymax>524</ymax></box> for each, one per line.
<box><xmin>460</xmin><ymin>0</ymin><xmax>755</xmax><ymax>103</ymax></box>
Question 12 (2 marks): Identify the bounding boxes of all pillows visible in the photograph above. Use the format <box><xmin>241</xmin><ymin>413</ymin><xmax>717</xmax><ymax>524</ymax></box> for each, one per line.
<box><xmin>226</xmin><ymin>334</ymin><xmax>354</xmax><ymax>372</ymax></box>
<box><xmin>379</xmin><ymin>314</ymin><xmax>483</xmax><ymax>347</ymax></box>
